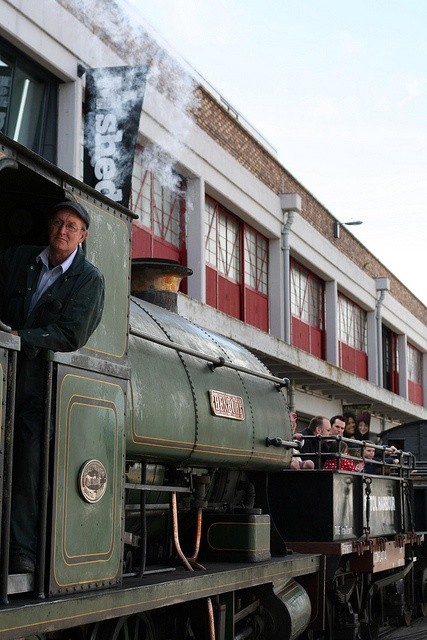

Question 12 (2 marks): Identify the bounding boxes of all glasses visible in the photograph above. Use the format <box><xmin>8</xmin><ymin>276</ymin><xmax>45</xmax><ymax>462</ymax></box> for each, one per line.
<box><xmin>52</xmin><ymin>218</ymin><xmax>85</xmax><ymax>233</ymax></box>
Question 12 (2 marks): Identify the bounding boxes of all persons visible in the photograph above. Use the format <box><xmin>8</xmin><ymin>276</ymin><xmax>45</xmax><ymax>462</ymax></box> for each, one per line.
<box><xmin>363</xmin><ymin>441</ymin><xmax>400</xmax><ymax>476</ymax></box>
<box><xmin>322</xmin><ymin>442</ymin><xmax>365</xmax><ymax>473</ymax></box>
<box><xmin>357</xmin><ymin>412</ymin><xmax>370</xmax><ymax>440</ymax></box>
<box><xmin>0</xmin><ymin>201</ymin><xmax>105</xmax><ymax>573</ymax></box>
<box><xmin>298</xmin><ymin>416</ymin><xmax>332</xmax><ymax>470</ymax></box>
<box><xmin>344</xmin><ymin>413</ymin><xmax>356</xmax><ymax>438</ymax></box>
<box><xmin>289</xmin><ymin>411</ymin><xmax>315</xmax><ymax>469</ymax></box>
<box><xmin>329</xmin><ymin>415</ymin><xmax>346</xmax><ymax>439</ymax></box>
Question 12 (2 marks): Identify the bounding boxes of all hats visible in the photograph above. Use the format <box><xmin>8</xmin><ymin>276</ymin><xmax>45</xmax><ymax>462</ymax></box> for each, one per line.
<box><xmin>53</xmin><ymin>200</ymin><xmax>91</xmax><ymax>228</ymax></box>
<box><xmin>356</xmin><ymin>411</ymin><xmax>371</xmax><ymax>424</ymax></box>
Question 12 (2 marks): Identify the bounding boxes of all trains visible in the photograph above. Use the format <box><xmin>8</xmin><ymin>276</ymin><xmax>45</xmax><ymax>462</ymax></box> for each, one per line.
<box><xmin>0</xmin><ymin>130</ymin><xmax>426</xmax><ymax>638</ymax></box>
